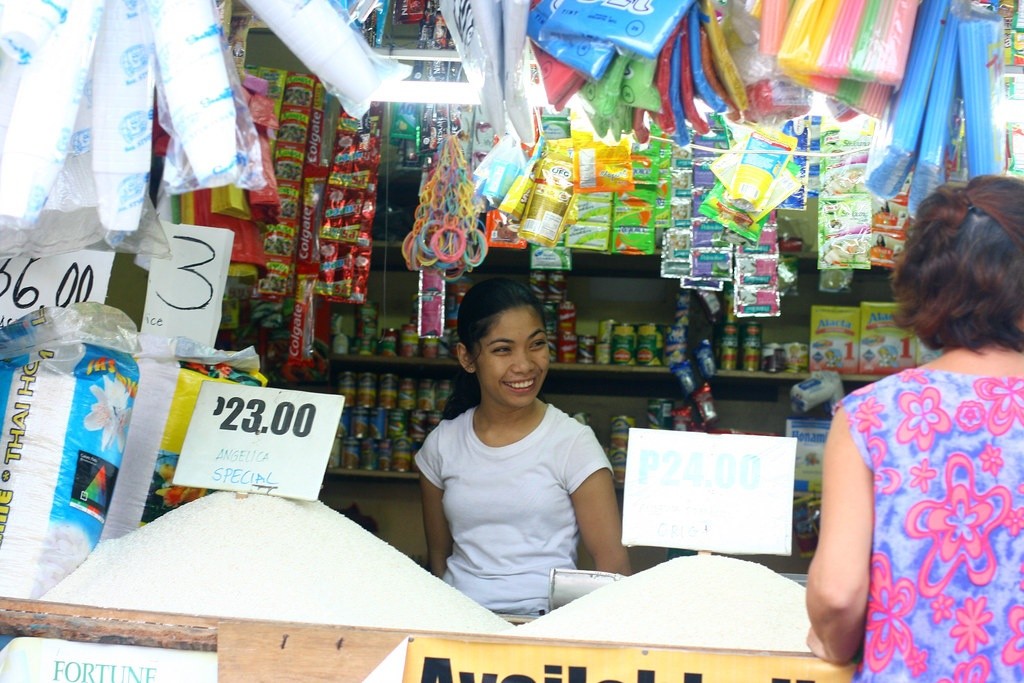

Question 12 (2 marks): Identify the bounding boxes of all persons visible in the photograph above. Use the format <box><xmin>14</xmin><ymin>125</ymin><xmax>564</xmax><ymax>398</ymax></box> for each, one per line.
<box><xmin>806</xmin><ymin>175</ymin><xmax>1024</xmax><ymax>683</ymax></box>
<box><xmin>413</xmin><ymin>277</ymin><xmax>629</xmax><ymax>615</ymax></box>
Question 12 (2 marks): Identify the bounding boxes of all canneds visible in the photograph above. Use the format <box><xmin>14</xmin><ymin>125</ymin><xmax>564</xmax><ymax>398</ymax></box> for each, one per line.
<box><xmin>326</xmin><ymin>292</ymin><xmax>466</xmax><ymax>472</ymax></box>
<box><xmin>574</xmin><ymin>398</ymin><xmax>695</xmax><ymax>485</ymax></box>
<box><xmin>528</xmin><ymin>268</ymin><xmax>811</xmax><ymax>372</ymax></box>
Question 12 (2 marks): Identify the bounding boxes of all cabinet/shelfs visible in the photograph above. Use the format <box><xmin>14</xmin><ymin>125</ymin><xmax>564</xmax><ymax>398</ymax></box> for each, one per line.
<box><xmin>303</xmin><ymin>237</ymin><xmax>886</xmax><ymax>495</ymax></box>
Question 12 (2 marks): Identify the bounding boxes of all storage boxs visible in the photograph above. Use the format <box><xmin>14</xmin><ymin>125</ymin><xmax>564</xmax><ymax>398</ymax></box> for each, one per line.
<box><xmin>860</xmin><ymin>301</ymin><xmax>916</xmax><ymax>376</ymax></box>
<box><xmin>809</xmin><ymin>305</ymin><xmax>861</xmax><ymax>374</ymax></box>
